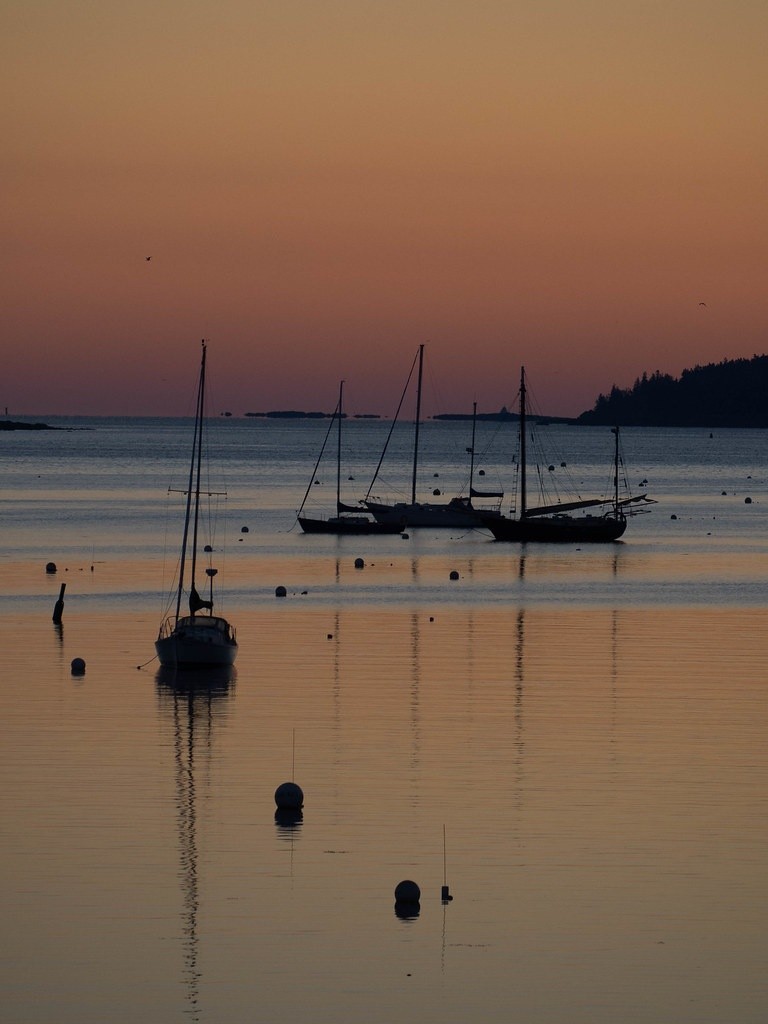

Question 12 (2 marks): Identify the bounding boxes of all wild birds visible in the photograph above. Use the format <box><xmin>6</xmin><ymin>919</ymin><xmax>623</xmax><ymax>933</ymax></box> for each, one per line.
<box><xmin>699</xmin><ymin>303</ymin><xmax>706</xmax><ymax>305</ymax></box>
<box><xmin>146</xmin><ymin>257</ymin><xmax>151</xmax><ymax>260</ymax></box>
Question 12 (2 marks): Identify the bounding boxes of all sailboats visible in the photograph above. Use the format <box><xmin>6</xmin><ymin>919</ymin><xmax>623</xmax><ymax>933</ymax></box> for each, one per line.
<box><xmin>155</xmin><ymin>344</ymin><xmax>238</xmax><ymax>679</ymax></box>
<box><xmin>472</xmin><ymin>364</ymin><xmax>626</xmax><ymax>543</ymax></box>
<box><xmin>296</xmin><ymin>378</ymin><xmax>406</xmax><ymax>535</ymax></box>
<box><xmin>362</xmin><ymin>344</ymin><xmax>506</xmax><ymax>524</ymax></box>
